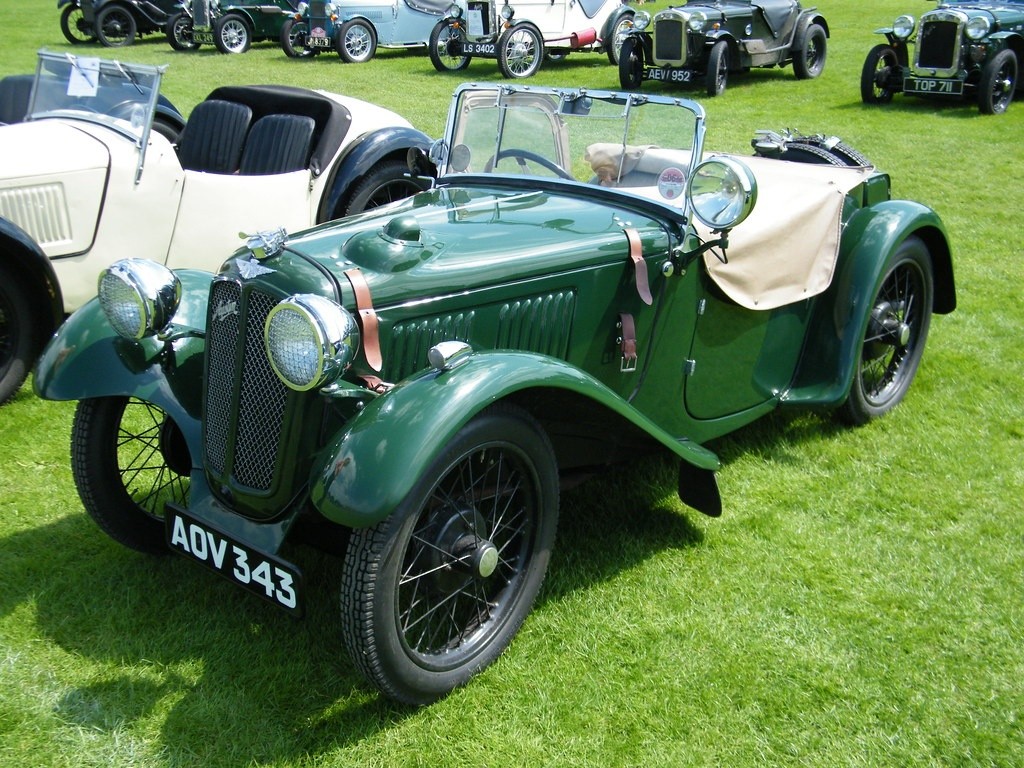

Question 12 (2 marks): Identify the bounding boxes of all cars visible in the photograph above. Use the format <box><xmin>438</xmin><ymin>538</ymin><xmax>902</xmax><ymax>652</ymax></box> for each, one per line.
<box><xmin>0</xmin><ymin>50</ymin><xmax>469</xmax><ymax>409</ymax></box>
<box><xmin>56</xmin><ymin>1</ymin><xmax>637</xmax><ymax>80</ymax></box>
<box><xmin>619</xmin><ymin>1</ymin><xmax>831</xmax><ymax>96</ymax></box>
<box><xmin>32</xmin><ymin>82</ymin><xmax>957</xmax><ymax>708</ymax></box>
<box><xmin>861</xmin><ymin>0</ymin><xmax>1023</xmax><ymax>114</ymax></box>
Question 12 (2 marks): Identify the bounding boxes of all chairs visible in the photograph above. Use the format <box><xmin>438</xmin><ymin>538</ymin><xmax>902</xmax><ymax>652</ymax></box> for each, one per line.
<box><xmin>179</xmin><ymin>100</ymin><xmax>253</xmax><ymax>172</ymax></box>
<box><xmin>238</xmin><ymin>113</ymin><xmax>316</xmax><ymax>174</ymax></box>
